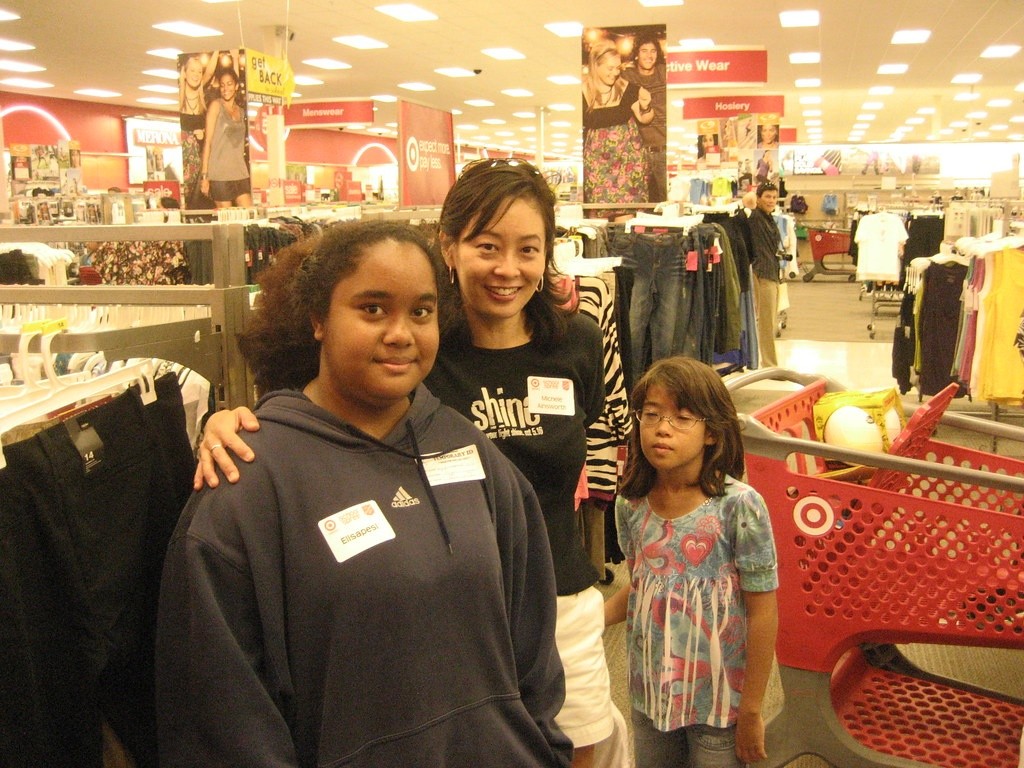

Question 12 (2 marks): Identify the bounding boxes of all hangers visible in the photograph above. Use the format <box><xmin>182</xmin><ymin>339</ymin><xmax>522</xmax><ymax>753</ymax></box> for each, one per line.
<box><xmin>549</xmin><ymin>199</ymin><xmax>745</xmax><ymax>277</ymax></box>
<box><xmin>853</xmin><ymin>202</ymin><xmax>1024</xmax><ymax>291</ymax></box>
<box><xmin>0</xmin><ymin>205</ymin><xmax>444</xmax><ymax>473</ymax></box>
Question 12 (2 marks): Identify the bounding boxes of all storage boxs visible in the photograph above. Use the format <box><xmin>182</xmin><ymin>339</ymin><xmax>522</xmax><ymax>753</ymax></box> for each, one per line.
<box><xmin>812</xmin><ymin>388</ymin><xmax>907</xmax><ymax>459</ymax></box>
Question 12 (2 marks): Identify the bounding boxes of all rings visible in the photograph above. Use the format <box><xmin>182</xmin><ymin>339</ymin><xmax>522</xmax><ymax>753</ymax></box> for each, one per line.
<box><xmin>210</xmin><ymin>444</ymin><xmax>221</xmax><ymax>451</ymax></box>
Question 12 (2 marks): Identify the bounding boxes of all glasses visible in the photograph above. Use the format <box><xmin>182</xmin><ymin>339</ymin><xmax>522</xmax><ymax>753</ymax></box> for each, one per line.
<box><xmin>453</xmin><ymin>158</ymin><xmax>540</xmax><ymax>191</ymax></box>
<box><xmin>763</xmin><ymin>182</ymin><xmax>774</xmax><ymax>187</ymax></box>
<box><xmin>636</xmin><ymin>408</ymin><xmax>710</xmax><ymax>429</ymax></box>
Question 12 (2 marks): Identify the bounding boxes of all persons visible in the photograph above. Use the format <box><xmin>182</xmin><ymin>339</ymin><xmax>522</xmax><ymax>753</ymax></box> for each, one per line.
<box><xmin>194</xmin><ymin>159</ymin><xmax>614</xmax><ymax>768</ymax></box>
<box><xmin>745</xmin><ymin>119</ymin><xmax>752</xmax><ymax>137</ymax></box>
<box><xmin>70</xmin><ymin>149</ymin><xmax>81</xmax><ymax>168</ymax></box>
<box><xmin>738</xmin><ymin>149</ymin><xmax>773</xmax><ymax>191</ymax></box>
<box><xmin>156</xmin><ymin>154</ymin><xmax>164</xmax><ymax>171</ymax></box>
<box><xmin>582</xmin><ymin>32</ymin><xmax>666</xmax><ymax>223</ymax></box>
<box><xmin>158</xmin><ymin>223</ymin><xmax>576</xmax><ymax>768</ymax></box>
<box><xmin>179</xmin><ymin>50</ymin><xmax>252</xmax><ymax>209</ymax></box>
<box><xmin>748</xmin><ymin>182</ymin><xmax>782</xmax><ymax>368</ymax></box>
<box><xmin>697</xmin><ymin>134</ymin><xmax>718</xmax><ymax>159</ymax></box>
<box><xmin>604</xmin><ymin>356</ymin><xmax>784</xmax><ymax>768</ymax></box>
<box><xmin>757</xmin><ymin>124</ymin><xmax>779</xmax><ymax>148</ymax></box>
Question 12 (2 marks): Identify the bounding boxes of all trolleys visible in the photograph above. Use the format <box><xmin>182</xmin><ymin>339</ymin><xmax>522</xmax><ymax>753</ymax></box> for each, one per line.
<box><xmin>724</xmin><ymin>367</ymin><xmax>1024</xmax><ymax>768</ymax></box>
<box><xmin>802</xmin><ymin>230</ymin><xmax>856</xmax><ymax>283</ymax></box>
